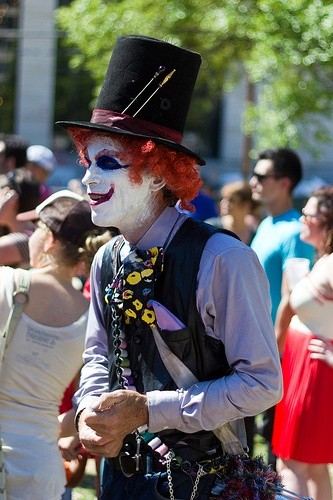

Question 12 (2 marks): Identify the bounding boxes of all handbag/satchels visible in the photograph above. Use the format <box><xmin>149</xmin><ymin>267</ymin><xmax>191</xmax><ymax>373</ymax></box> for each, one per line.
<box><xmin>0</xmin><ymin>439</ymin><xmax>7</xmax><ymax>500</ymax></box>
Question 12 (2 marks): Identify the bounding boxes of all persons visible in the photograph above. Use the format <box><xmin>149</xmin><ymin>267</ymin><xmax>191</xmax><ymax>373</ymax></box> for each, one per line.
<box><xmin>53</xmin><ymin>36</ymin><xmax>283</xmax><ymax>500</ymax></box>
<box><xmin>0</xmin><ymin>133</ymin><xmax>118</xmax><ymax>500</ymax></box>
<box><xmin>177</xmin><ymin>148</ymin><xmax>333</xmax><ymax>500</ymax></box>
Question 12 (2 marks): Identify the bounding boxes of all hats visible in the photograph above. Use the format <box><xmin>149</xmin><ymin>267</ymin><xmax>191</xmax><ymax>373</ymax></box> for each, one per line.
<box><xmin>55</xmin><ymin>34</ymin><xmax>207</xmax><ymax>166</ymax></box>
<box><xmin>15</xmin><ymin>189</ymin><xmax>96</xmax><ymax>244</ymax></box>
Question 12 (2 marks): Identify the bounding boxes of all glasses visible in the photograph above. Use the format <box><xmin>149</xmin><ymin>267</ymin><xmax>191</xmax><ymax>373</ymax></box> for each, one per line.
<box><xmin>301</xmin><ymin>208</ymin><xmax>317</xmax><ymax>222</ymax></box>
<box><xmin>251</xmin><ymin>172</ymin><xmax>283</xmax><ymax>182</ymax></box>
